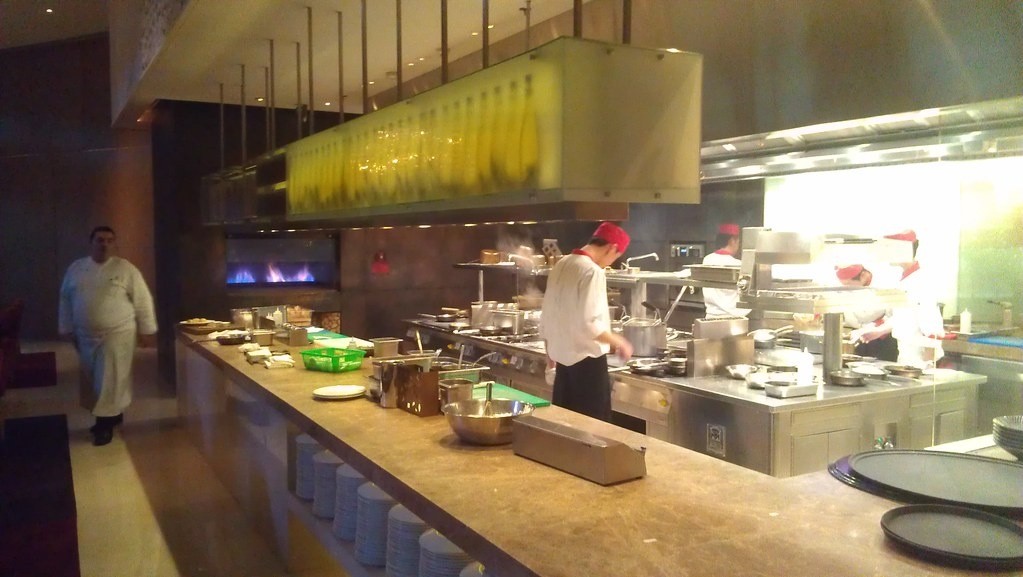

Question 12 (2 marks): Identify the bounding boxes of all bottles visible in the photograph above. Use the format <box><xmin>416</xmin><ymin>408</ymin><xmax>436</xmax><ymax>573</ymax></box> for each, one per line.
<box><xmin>960</xmin><ymin>308</ymin><xmax>971</xmax><ymax>334</ymax></box>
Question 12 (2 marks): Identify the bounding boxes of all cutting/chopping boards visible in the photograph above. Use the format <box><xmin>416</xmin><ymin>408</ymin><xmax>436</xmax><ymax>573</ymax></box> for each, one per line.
<box><xmin>177</xmin><ymin>322</ymin><xmax>245</xmax><ymax>335</ymax></box>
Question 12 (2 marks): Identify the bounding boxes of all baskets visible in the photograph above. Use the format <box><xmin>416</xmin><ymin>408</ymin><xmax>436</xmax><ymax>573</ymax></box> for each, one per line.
<box><xmin>300</xmin><ymin>346</ymin><xmax>366</xmax><ymax>373</ymax></box>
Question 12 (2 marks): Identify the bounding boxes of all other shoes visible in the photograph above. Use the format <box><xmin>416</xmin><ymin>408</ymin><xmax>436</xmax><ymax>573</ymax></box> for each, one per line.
<box><xmin>89</xmin><ymin>412</ymin><xmax>124</xmax><ymax>444</ymax></box>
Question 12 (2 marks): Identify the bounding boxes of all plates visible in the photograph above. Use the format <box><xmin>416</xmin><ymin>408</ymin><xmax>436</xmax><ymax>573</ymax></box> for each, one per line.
<box><xmin>312</xmin><ymin>385</ymin><xmax>367</xmax><ymax>400</ymax></box>
<box><xmin>179</xmin><ymin>320</ymin><xmax>215</xmax><ymax>324</ymax></box>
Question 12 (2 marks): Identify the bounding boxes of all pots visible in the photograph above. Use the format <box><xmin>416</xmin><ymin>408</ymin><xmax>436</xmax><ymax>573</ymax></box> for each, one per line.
<box><xmin>747</xmin><ymin>325</ymin><xmax>794</xmax><ymax>349</ymax></box>
<box><xmin>493</xmin><ymin>309</ymin><xmax>524</xmax><ymax>335</ymax></box>
<box><xmin>622</xmin><ymin>321</ymin><xmax>667</xmax><ymax>359</ymax></box>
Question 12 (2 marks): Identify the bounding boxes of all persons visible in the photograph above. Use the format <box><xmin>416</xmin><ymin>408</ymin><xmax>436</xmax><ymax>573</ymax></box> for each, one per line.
<box><xmin>58</xmin><ymin>224</ymin><xmax>156</xmax><ymax>445</ymax></box>
<box><xmin>701</xmin><ymin>223</ymin><xmax>755</xmax><ymax>318</ymax></box>
<box><xmin>826</xmin><ymin>226</ymin><xmax>944</xmax><ymax>370</ymax></box>
<box><xmin>538</xmin><ymin>222</ymin><xmax>635</xmax><ymax>423</ymax></box>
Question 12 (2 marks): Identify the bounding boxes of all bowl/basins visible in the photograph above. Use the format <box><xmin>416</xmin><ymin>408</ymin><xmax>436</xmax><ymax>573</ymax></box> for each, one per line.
<box><xmin>668</xmin><ymin>358</ymin><xmax>688</xmax><ymax>375</ymax></box>
<box><xmin>440</xmin><ymin>397</ymin><xmax>536</xmax><ymax>446</ymax></box>
<box><xmin>295</xmin><ymin>433</ymin><xmax>493</xmax><ymax>577</ymax></box>
<box><xmin>993</xmin><ymin>416</ymin><xmax>1023</xmax><ymax>460</ymax></box>
<box><xmin>436</xmin><ymin>314</ymin><xmax>457</xmax><ymax>322</ymax></box>
<box><xmin>217</xmin><ymin>334</ymin><xmax>247</xmax><ymax>345</ymax></box>
<box><xmin>831</xmin><ymin>370</ymin><xmax>865</xmax><ymax>386</ymax></box>
<box><xmin>627</xmin><ymin>359</ymin><xmax>662</xmax><ymax>374</ymax></box>
<box><xmin>479</xmin><ymin>326</ymin><xmax>502</xmax><ymax>336</ymax></box>
<box><xmin>726</xmin><ymin>365</ymin><xmax>757</xmax><ymax>380</ymax></box>
<box><xmin>448</xmin><ymin>323</ymin><xmax>471</xmax><ymax>331</ymax></box>
<box><xmin>844</xmin><ymin>362</ymin><xmax>923</xmax><ymax>379</ymax></box>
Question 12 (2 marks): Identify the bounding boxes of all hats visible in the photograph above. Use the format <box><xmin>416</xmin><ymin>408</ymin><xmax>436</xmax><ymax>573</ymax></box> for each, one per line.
<box><xmin>834</xmin><ymin>264</ymin><xmax>862</xmax><ymax>280</ymax></box>
<box><xmin>593</xmin><ymin>220</ymin><xmax>631</xmax><ymax>253</ymax></box>
<box><xmin>719</xmin><ymin>223</ymin><xmax>739</xmax><ymax>234</ymax></box>
<box><xmin>884</xmin><ymin>229</ymin><xmax>917</xmax><ymax>243</ymax></box>
